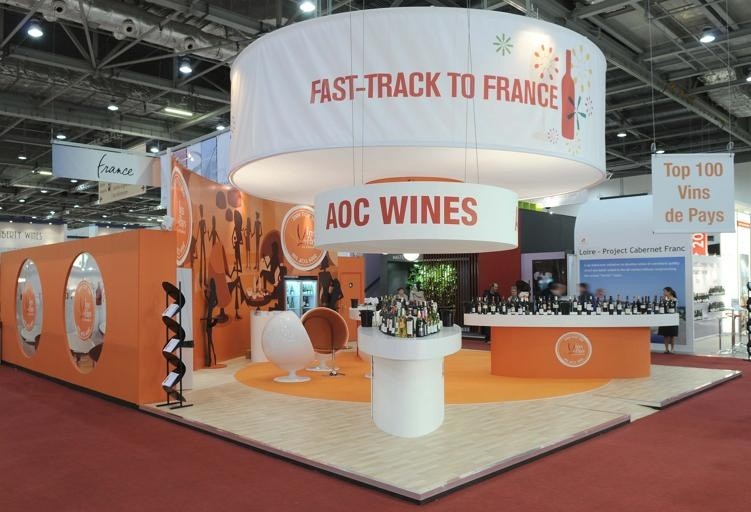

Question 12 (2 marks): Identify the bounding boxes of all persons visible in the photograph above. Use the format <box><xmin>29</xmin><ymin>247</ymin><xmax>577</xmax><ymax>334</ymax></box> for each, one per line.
<box><xmin>658</xmin><ymin>286</ymin><xmax>679</xmax><ymax>355</ymax></box>
<box><xmin>393</xmin><ymin>288</ymin><xmax>409</xmax><ymax>303</ymax></box>
<box><xmin>535</xmin><ymin>260</ymin><xmax>553</xmax><ymax>290</ymax></box>
<box><xmin>508</xmin><ymin>285</ymin><xmax>519</xmax><ymax>302</ymax></box>
<box><xmin>593</xmin><ymin>288</ymin><xmax>607</xmax><ymax>306</ymax></box>
<box><xmin>546</xmin><ymin>283</ymin><xmax>566</xmax><ymax>312</ymax></box>
<box><xmin>482</xmin><ymin>282</ymin><xmax>502</xmax><ymax>345</ymax></box>
<box><xmin>577</xmin><ymin>283</ymin><xmax>596</xmax><ymax>309</ymax></box>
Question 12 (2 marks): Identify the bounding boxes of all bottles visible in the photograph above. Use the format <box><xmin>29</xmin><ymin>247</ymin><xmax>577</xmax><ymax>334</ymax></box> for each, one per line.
<box><xmin>290</xmin><ymin>297</ymin><xmax>294</xmax><ymax>308</ymax></box>
<box><xmin>470</xmin><ymin>294</ymin><xmax>676</xmax><ymax>315</ymax></box>
<box><xmin>303</xmin><ymin>296</ymin><xmax>309</xmax><ymax>307</ymax></box>
<box><xmin>290</xmin><ymin>285</ymin><xmax>294</xmax><ymax>295</ymax></box>
<box><xmin>379</xmin><ymin>294</ymin><xmax>441</xmax><ymax>338</ymax></box>
<box><xmin>302</xmin><ymin>284</ymin><xmax>313</xmax><ymax>295</ymax></box>
<box><xmin>95</xmin><ymin>282</ymin><xmax>102</xmax><ymax>306</ymax></box>
<box><xmin>287</xmin><ymin>296</ymin><xmax>289</xmax><ymax>308</ymax></box>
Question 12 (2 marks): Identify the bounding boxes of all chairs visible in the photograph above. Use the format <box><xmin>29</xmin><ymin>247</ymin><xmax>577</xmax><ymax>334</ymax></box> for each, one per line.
<box><xmin>260</xmin><ymin>305</ymin><xmax>350</xmax><ymax>385</ymax></box>
